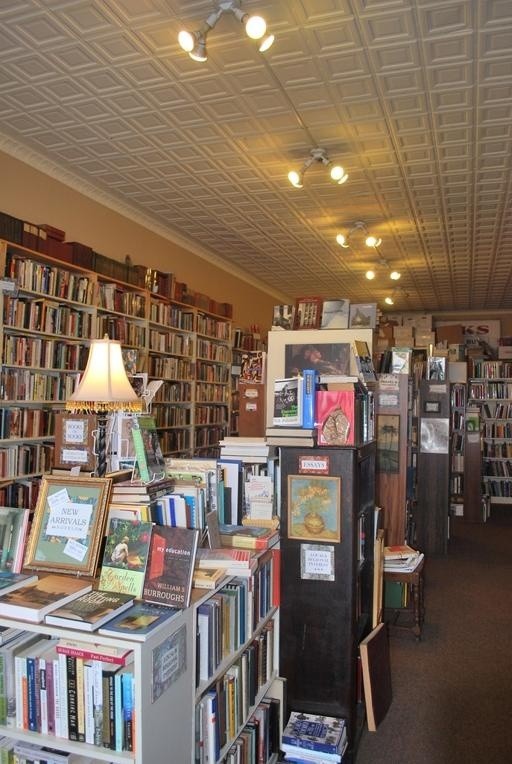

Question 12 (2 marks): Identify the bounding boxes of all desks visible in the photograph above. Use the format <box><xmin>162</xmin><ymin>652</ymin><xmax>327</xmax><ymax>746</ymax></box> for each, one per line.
<box><xmin>383</xmin><ymin>552</ymin><xmax>428</xmax><ymax>643</ymax></box>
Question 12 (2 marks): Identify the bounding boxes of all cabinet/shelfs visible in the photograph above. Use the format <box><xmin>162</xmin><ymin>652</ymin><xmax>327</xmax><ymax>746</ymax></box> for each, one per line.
<box><xmin>2</xmin><ymin>240</ymin><xmax>150</xmax><ymax>513</ymax></box>
<box><xmin>145</xmin><ymin>268</ymin><xmax>266</xmax><ymax>461</ymax></box>
<box><xmin>195</xmin><ymin>538</ymin><xmax>279</xmax><ymax>762</ymax></box>
<box><xmin>279</xmin><ymin>441</ymin><xmax>378</xmax><ymax>760</ymax></box>
<box><xmin>373</xmin><ymin>347</ymin><xmax>512</xmax><ymax>557</ymax></box>
<box><xmin>1</xmin><ymin>609</ymin><xmax>196</xmax><ymax>763</ymax></box>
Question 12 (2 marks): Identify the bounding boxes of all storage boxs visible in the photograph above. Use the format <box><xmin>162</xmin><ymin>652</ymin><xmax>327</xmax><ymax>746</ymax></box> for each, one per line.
<box><xmin>322</xmin><ymin>299</ymin><xmax>471</xmax><ymax>385</ymax></box>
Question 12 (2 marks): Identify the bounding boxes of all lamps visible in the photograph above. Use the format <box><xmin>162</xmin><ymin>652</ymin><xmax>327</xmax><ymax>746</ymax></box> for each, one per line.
<box><xmin>364</xmin><ymin>258</ymin><xmax>404</xmax><ymax>282</ymax></box>
<box><xmin>174</xmin><ymin>1</ymin><xmax>279</xmax><ymax>64</ymax></box>
<box><xmin>286</xmin><ymin>144</ymin><xmax>349</xmax><ymax>189</ymax></box>
<box><xmin>384</xmin><ymin>286</ymin><xmax>411</xmax><ymax>306</ymax></box>
<box><xmin>334</xmin><ymin>218</ymin><xmax>383</xmax><ymax>249</ymax></box>
<box><xmin>65</xmin><ymin>336</ymin><xmax>144</xmax><ymax>478</ymax></box>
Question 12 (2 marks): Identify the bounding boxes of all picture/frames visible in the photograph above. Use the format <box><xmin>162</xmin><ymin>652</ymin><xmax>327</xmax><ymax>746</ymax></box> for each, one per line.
<box><xmin>17</xmin><ymin>474</ymin><xmax>112</xmax><ymax>582</ymax></box>
<box><xmin>293</xmin><ymin>295</ymin><xmax>321</xmax><ymax>331</ymax></box>
